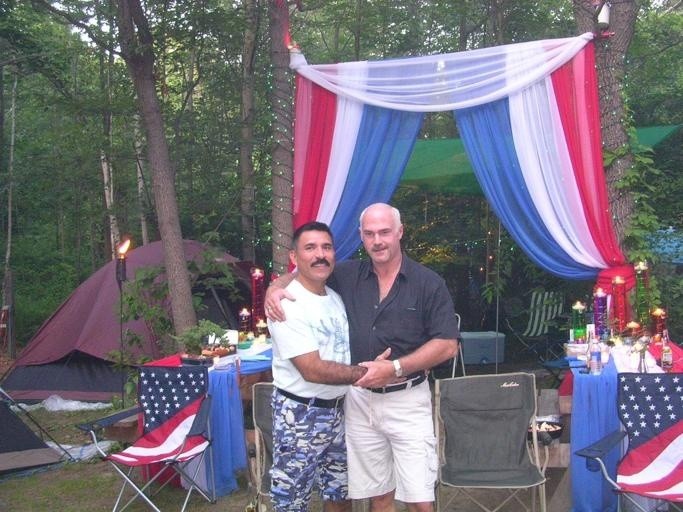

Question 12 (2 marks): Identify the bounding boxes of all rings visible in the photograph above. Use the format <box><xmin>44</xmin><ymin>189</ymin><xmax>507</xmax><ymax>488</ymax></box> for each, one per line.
<box><xmin>267</xmin><ymin>306</ymin><xmax>272</xmax><ymax>313</ymax></box>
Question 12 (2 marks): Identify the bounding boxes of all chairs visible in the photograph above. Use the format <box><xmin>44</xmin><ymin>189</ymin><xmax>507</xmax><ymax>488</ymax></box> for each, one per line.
<box><xmin>252</xmin><ymin>382</ymin><xmax>272</xmax><ymax>512</ymax></box>
<box><xmin>76</xmin><ymin>365</ymin><xmax>213</xmax><ymax>512</ymax></box>
<box><xmin>505</xmin><ymin>290</ymin><xmax>566</xmax><ymax>361</ymax></box>
<box><xmin>431</xmin><ymin>313</ymin><xmax>465</xmax><ymax>379</ymax></box>
<box><xmin>434</xmin><ymin>372</ymin><xmax>552</xmax><ymax>512</ymax></box>
<box><xmin>538</xmin><ymin>332</ymin><xmax>570</xmax><ymax>388</ymax></box>
<box><xmin>574</xmin><ymin>373</ymin><xmax>683</xmax><ymax>512</ymax></box>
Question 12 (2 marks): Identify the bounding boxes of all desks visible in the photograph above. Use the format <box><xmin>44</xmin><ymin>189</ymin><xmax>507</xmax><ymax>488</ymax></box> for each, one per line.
<box><xmin>145</xmin><ymin>341</ymin><xmax>271</xmax><ymax>498</ymax></box>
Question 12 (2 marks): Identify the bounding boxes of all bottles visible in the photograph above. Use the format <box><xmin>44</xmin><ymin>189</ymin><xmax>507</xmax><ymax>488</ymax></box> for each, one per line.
<box><xmin>571</xmin><ymin>299</ymin><xmax>586</xmax><ymax>343</ymax></box>
<box><xmin>255</xmin><ymin>320</ymin><xmax>267</xmax><ymax>339</ymax></box>
<box><xmin>590</xmin><ymin>340</ymin><xmax>601</xmax><ymax>377</ymax></box>
<box><xmin>660</xmin><ymin>329</ymin><xmax>673</xmax><ymax>368</ymax></box>
<box><xmin>585</xmin><ymin>323</ymin><xmax>595</xmax><ymax>368</ymax></box>
<box><xmin>237</xmin><ymin>307</ymin><xmax>251</xmax><ymax>335</ymax></box>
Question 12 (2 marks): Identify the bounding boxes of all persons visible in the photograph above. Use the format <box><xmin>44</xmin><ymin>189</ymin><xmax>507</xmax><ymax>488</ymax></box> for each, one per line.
<box><xmin>264</xmin><ymin>202</ymin><xmax>462</xmax><ymax>512</ymax></box>
<box><xmin>267</xmin><ymin>222</ymin><xmax>411</xmax><ymax>512</ymax></box>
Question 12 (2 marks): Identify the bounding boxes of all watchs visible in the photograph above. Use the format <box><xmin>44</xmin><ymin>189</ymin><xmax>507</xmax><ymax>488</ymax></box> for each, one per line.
<box><xmin>393</xmin><ymin>359</ymin><xmax>403</xmax><ymax>379</ymax></box>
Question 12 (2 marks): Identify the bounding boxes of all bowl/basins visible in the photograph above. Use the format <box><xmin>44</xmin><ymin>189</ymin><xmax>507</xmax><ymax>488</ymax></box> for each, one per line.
<box><xmin>527</xmin><ymin>420</ymin><xmax>565</xmax><ymax>440</ymax></box>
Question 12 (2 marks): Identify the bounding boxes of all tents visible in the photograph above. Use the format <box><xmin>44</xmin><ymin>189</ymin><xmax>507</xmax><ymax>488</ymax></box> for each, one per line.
<box><xmin>1</xmin><ymin>234</ymin><xmax>269</xmax><ymax>409</ymax></box>
<box><xmin>0</xmin><ymin>386</ymin><xmax>76</xmax><ymax>482</ymax></box>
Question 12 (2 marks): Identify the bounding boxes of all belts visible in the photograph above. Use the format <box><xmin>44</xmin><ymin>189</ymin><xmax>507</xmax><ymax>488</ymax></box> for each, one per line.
<box><xmin>367</xmin><ymin>376</ymin><xmax>426</xmax><ymax>393</ymax></box>
<box><xmin>277</xmin><ymin>388</ymin><xmax>345</xmax><ymax>408</ymax></box>
